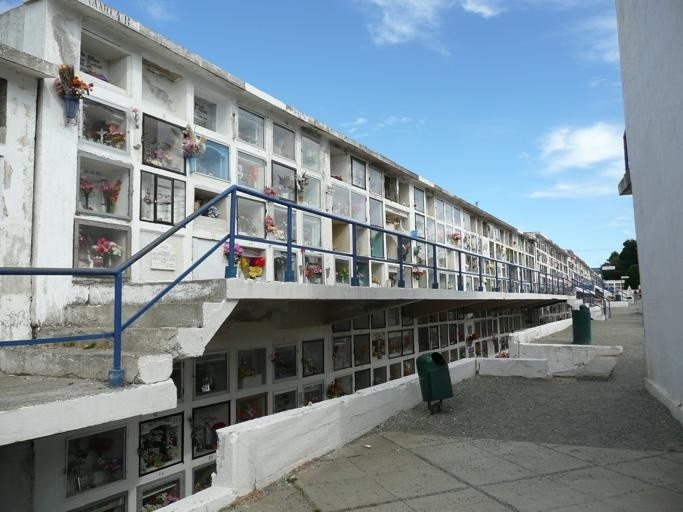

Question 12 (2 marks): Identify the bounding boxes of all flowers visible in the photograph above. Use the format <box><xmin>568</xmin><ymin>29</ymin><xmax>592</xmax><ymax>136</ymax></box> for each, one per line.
<box><xmin>53</xmin><ymin>64</ymin><xmax>131</xmax><ymax>264</ymax></box>
<box><xmin>223</xmin><ymin>162</ymin><xmax>323</xmax><ymax>281</ymax></box>
<box><xmin>149</xmin><ymin>123</ymin><xmax>207</xmax><ymax>165</ymax></box>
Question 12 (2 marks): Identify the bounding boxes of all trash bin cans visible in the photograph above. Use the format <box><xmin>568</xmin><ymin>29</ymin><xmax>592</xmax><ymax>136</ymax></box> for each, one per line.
<box><xmin>416</xmin><ymin>352</ymin><xmax>453</xmax><ymax>415</ymax></box>
<box><xmin>572</xmin><ymin>305</ymin><xmax>594</xmax><ymax>345</ymax></box>
<box><xmin>615</xmin><ymin>295</ymin><xmax>621</xmax><ymax>301</ymax></box>
